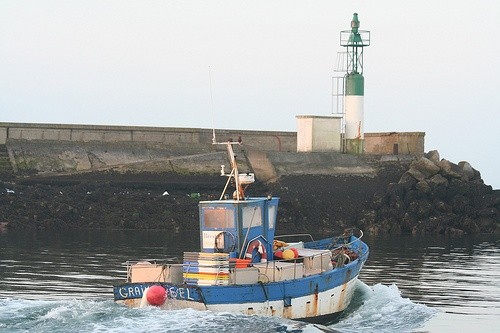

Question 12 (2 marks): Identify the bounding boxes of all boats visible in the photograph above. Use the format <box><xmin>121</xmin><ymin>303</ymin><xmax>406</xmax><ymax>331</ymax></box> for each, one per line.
<box><xmin>113</xmin><ymin>160</ymin><xmax>370</xmax><ymax>324</ymax></box>
